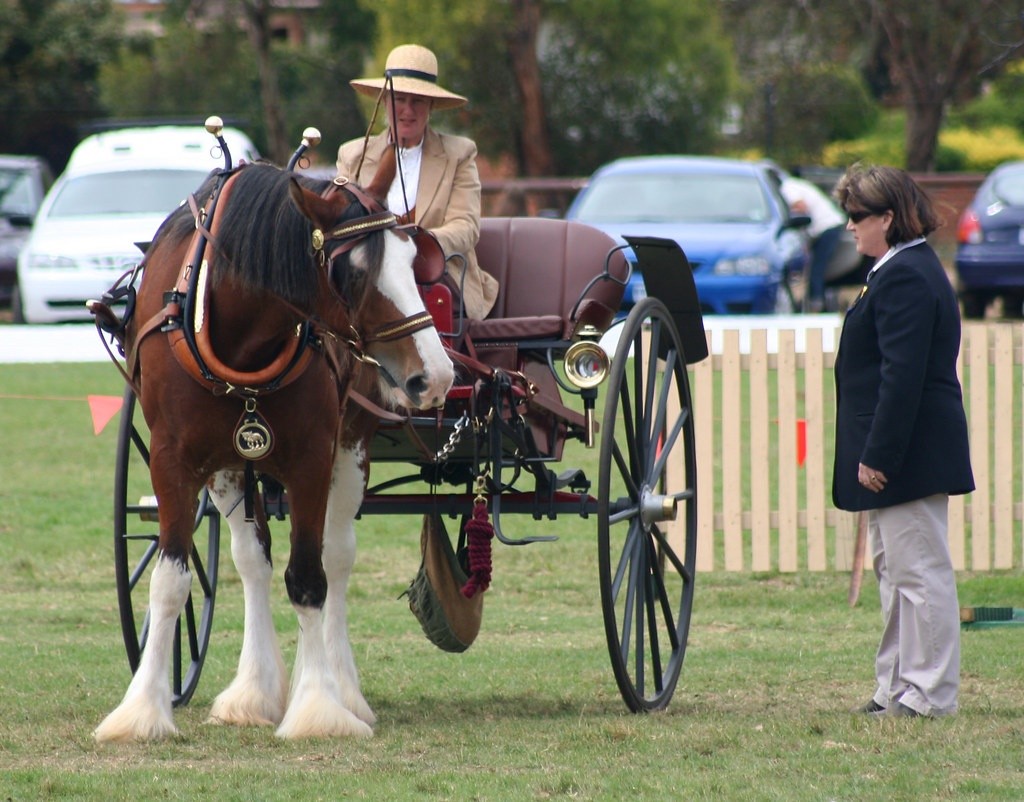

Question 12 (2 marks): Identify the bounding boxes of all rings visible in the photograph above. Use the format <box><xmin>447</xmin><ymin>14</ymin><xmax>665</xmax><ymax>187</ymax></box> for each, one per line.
<box><xmin>872</xmin><ymin>476</ymin><xmax>875</xmax><ymax>480</ymax></box>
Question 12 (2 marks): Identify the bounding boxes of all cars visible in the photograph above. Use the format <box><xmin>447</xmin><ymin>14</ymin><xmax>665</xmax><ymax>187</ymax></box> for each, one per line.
<box><xmin>762</xmin><ymin>154</ymin><xmax>876</xmax><ymax>292</ymax></box>
<box><xmin>16</xmin><ymin>120</ymin><xmax>261</xmax><ymax>328</ymax></box>
<box><xmin>954</xmin><ymin>163</ymin><xmax>1024</xmax><ymax>321</ymax></box>
<box><xmin>531</xmin><ymin>153</ymin><xmax>816</xmax><ymax>324</ymax></box>
<box><xmin>1</xmin><ymin>154</ymin><xmax>54</xmax><ymax>324</ymax></box>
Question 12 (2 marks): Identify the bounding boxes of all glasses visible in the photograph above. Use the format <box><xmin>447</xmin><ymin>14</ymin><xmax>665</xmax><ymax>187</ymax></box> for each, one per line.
<box><xmin>846</xmin><ymin>205</ymin><xmax>893</xmax><ymax>224</ymax></box>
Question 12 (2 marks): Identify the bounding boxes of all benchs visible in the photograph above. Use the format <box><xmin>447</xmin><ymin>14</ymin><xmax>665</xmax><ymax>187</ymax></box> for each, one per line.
<box><xmin>461</xmin><ymin>218</ymin><xmax>627</xmax><ymax>350</ymax></box>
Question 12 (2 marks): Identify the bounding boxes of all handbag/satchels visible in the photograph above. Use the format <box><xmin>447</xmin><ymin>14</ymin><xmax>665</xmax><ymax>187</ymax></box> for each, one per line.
<box><xmin>408</xmin><ymin>509</ymin><xmax>484</xmax><ymax>653</ymax></box>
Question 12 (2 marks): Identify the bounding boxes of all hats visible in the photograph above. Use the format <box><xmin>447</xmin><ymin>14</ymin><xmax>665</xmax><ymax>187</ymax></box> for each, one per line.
<box><xmin>349</xmin><ymin>44</ymin><xmax>469</xmax><ymax>111</ymax></box>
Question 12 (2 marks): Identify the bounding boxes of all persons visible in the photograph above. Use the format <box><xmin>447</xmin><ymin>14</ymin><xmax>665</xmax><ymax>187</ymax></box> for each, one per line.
<box><xmin>832</xmin><ymin>167</ymin><xmax>975</xmax><ymax>720</ymax></box>
<box><xmin>780</xmin><ymin>176</ymin><xmax>845</xmax><ymax>309</ymax></box>
<box><xmin>336</xmin><ymin>45</ymin><xmax>500</xmax><ymax>320</ymax></box>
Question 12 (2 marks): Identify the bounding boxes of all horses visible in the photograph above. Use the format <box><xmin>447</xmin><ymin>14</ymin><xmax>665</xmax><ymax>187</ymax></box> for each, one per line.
<box><xmin>89</xmin><ymin>160</ymin><xmax>455</xmax><ymax>744</ymax></box>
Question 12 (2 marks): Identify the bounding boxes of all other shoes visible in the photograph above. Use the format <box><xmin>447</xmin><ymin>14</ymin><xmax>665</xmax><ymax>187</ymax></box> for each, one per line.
<box><xmin>850</xmin><ymin>698</ymin><xmax>885</xmax><ymax>716</ymax></box>
<box><xmin>869</xmin><ymin>702</ymin><xmax>933</xmax><ymax>721</ymax></box>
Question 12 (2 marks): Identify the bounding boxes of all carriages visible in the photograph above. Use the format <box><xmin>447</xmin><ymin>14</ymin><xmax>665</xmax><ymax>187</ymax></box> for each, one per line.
<box><xmin>86</xmin><ymin>109</ymin><xmax>701</xmax><ymax>714</ymax></box>
